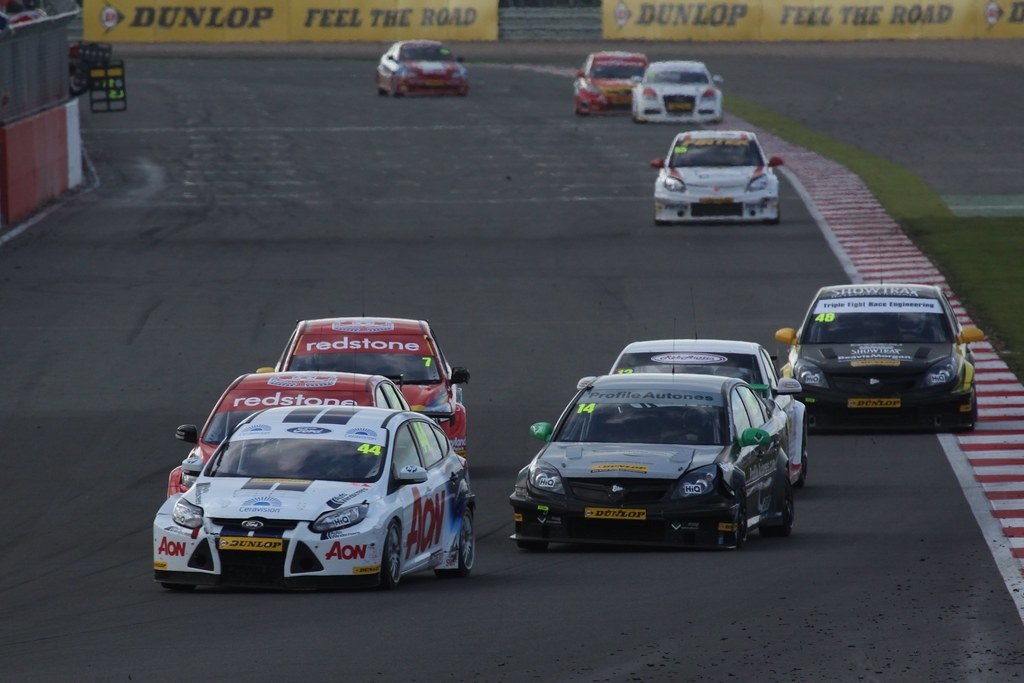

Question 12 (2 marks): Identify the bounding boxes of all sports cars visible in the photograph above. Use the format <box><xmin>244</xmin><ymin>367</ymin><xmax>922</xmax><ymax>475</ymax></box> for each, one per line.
<box><xmin>574</xmin><ymin>50</ymin><xmax>652</xmax><ymax>116</ymax></box>
<box><xmin>507</xmin><ymin>373</ymin><xmax>793</xmax><ymax>551</ymax></box>
<box><xmin>630</xmin><ymin>60</ymin><xmax>725</xmax><ymax>125</ymax></box>
<box><xmin>611</xmin><ymin>338</ymin><xmax>810</xmax><ymax>489</ymax></box>
<box><xmin>653</xmin><ymin>128</ymin><xmax>783</xmax><ymax>223</ymax></box>
<box><xmin>263</xmin><ymin>315</ymin><xmax>471</xmax><ymax>458</ymax></box>
<box><xmin>167</xmin><ymin>366</ymin><xmax>411</xmax><ymax>497</ymax></box>
<box><xmin>377</xmin><ymin>40</ymin><xmax>471</xmax><ymax>97</ymax></box>
<box><xmin>771</xmin><ymin>282</ymin><xmax>984</xmax><ymax>432</ymax></box>
<box><xmin>150</xmin><ymin>404</ymin><xmax>478</xmax><ymax>587</ymax></box>
